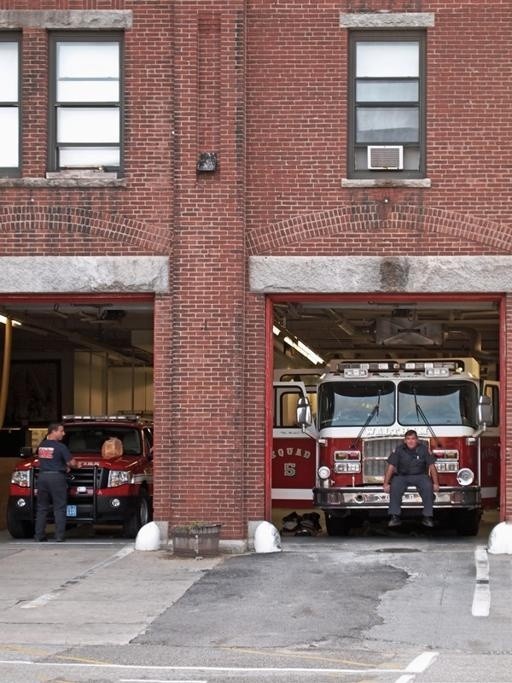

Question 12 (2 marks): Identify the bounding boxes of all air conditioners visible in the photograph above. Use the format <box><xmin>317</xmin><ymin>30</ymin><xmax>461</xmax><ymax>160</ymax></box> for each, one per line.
<box><xmin>368</xmin><ymin>146</ymin><xmax>404</xmax><ymax>170</ymax></box>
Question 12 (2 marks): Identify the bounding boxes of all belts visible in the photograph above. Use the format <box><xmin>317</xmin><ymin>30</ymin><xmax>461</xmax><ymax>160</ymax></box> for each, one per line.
<box><xmin>41</xmin><ymin>470</ymin><xmax>65</xmax><ymax>474</ymax></box>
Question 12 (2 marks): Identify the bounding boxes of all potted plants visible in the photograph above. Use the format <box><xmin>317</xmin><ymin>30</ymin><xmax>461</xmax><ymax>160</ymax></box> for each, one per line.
<box><xmin>172</xmin><ymin>521</ymin><xmax>223</xmax><ymax>557</ymax></box>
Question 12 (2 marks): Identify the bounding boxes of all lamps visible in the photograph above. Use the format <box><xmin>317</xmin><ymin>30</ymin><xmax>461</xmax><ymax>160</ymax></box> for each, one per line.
<box><xmin>272</xmin><ymin>325</ymin><xmax>318</xmax><ymax>366</ymax></box>
<box><xmin>0</xmin><ymin>310</ymin><xmax>26</xmax><ymax>327</ymax></box>
<box><xmin>294</xmin><ymin>338</ymin><xmax>328</xmax><ymax>366</ymax></box>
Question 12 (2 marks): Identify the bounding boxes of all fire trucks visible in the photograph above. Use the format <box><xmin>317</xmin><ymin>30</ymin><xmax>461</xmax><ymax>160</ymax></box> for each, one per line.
<box><xmin>270</xmin><ymin>357</ymin><xmax>501</xmax><ymax>538</ymax></box>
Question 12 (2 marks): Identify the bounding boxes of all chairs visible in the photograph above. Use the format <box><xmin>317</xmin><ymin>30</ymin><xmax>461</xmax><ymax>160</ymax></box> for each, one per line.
<box><xmin>123</xmin><ymin>432</ymin><xmax>141</xmax><ymax>451</ymax></box>
<box><xmin>69</xmin><ymin>432</ymin><xmax>88</xmax><ymax>450</ymax></box>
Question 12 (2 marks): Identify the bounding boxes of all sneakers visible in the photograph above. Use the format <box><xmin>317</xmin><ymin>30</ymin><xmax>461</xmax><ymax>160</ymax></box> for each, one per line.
<box><xmin>423</xmin><ymin>518</ymin><xmax>434</xmax><ymax>527</ymax></box>
<box><xmin>389</xmin><ymin>518</ymin><xmax>403</xmax><ymax>527</ymax></box>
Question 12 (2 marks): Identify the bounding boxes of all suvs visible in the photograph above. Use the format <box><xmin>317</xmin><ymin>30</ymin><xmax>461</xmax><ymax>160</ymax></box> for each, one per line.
<box><xmin>6</xmin><ymin>414</ymin><xmax>156</xmax><ymax>539</ymax></box>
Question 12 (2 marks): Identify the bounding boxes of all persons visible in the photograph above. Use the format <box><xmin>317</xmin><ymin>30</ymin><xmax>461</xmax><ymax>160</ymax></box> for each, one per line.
<box><xmin>382</xmin><ymin>428</ymin><xmax>440</xmax><ymax>528</ymax></box>
<box><xmin>34</xmin><ymin>422</ymin><xmax>76</xmax><ymax>542</ymax></box>
